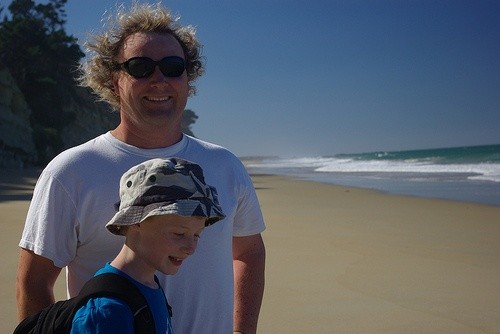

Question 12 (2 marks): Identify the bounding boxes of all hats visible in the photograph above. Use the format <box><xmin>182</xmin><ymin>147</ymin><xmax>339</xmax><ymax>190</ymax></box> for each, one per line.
<box><xmin>105</xmin><ymin>157</ymin><xmax>226</xmax><ymax>235</ymax></box>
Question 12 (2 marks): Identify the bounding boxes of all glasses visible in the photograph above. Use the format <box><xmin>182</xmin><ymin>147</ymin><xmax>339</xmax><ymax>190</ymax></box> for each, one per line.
<box><xmin>117</xmin><ymin>56</ymin><xmax>186</xmax><ymax>79</ymax></box>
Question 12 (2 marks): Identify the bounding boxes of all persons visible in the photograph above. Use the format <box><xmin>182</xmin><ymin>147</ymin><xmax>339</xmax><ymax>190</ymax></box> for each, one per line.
<box><xmin>69</xmin><ymin>158</ymin><xmax>225</xmax><ymax>334</ymax></box>
<box><xmin>17</xmin><ymin>0</ymin><xmax>266</xmax><ymax>334</ymax></box>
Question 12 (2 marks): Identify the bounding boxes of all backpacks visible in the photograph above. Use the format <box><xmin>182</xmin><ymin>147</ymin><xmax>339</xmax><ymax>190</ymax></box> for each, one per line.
<box><xmin>13</xmin><ymin>275</ymin><xmax>172</xmax><ymax>334</ymax></box>
